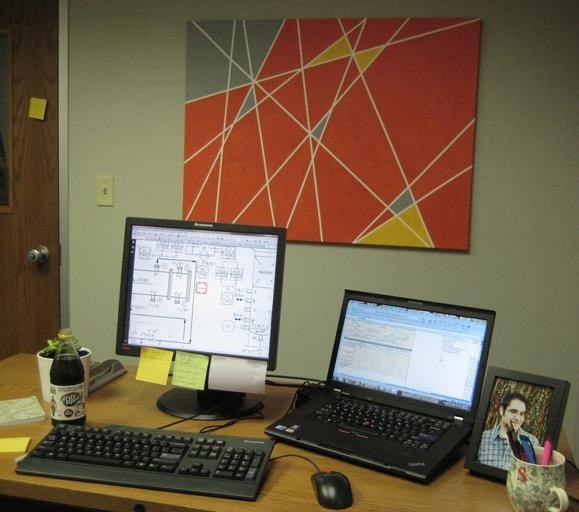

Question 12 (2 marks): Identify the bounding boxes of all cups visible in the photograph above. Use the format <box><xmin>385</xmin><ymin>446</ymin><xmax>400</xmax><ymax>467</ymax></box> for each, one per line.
<box><xmin>505</xmin><ymin>447</ymin><xmax>570</xmax><ymax>512</ymax></box>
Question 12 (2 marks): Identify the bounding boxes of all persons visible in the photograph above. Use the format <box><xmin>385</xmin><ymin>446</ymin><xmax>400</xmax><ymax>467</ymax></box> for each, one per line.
<box><xmin>475</xmin><ymin>393</ymin><xmax>540</xmax><ymax>472</ymax></box>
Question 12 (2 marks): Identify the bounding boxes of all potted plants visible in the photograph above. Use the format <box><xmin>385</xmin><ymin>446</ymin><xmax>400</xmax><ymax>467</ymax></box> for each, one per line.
<box><xmin>36</xmin><ymin>334</ymin><xmax>94</xmax><ymax>405</ymax></box>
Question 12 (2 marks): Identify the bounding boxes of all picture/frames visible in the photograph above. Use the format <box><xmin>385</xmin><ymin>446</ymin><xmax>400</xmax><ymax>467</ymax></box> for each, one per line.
<box><xmin>462</xmin><ymin>364</ymin><xmax>571</xmax><ymax>482</ymax></box>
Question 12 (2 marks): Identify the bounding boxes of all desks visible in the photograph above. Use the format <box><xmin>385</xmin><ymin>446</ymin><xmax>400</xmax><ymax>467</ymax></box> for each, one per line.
<box><xmin>0</xmin><ymin>351</ymin><xmax>579</xmax><ymax>512</ymax></box>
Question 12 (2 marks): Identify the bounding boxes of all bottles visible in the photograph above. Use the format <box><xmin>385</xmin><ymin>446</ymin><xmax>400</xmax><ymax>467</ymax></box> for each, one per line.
<box><xmin>50</xmin><ymin>330</ymin><xmax>87</xmax><ymax>428</ymax></box>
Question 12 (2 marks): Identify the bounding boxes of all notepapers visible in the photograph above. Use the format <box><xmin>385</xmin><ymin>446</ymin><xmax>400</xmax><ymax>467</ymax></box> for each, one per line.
<box><xmin>0</xmin><ymin>437</ymin><xmax>31</xmax><ymax>454</ymax></box>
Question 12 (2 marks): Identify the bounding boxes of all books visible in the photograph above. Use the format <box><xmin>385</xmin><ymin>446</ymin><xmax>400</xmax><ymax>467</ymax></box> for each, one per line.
<box><xmin>0</xmin><ymin>395</ymin><xmax>47</xmax><ymax>428</ymax></box>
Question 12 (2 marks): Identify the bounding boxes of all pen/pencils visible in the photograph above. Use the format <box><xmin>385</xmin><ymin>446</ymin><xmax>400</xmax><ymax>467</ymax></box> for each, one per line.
<box><xmin>506</xmin><ymin>420</ymin><xmax>553</xmax><ymax>465</ymax></box>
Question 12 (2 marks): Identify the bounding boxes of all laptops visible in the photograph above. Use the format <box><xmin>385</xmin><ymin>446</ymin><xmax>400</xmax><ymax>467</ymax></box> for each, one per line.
<box><xmin>263</xmin><ymin>287</ymin><xmax>497</xmax><ymax>486</ymax></box>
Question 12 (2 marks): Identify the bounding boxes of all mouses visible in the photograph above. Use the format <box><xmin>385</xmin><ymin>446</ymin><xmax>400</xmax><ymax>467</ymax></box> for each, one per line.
<box><xmin>309</xmin><ymin>468</ymin><xmax>354</xmax><ymax>510</ymax></box>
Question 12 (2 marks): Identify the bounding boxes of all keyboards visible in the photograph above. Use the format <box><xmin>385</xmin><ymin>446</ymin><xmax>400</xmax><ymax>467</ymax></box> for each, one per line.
<box><xmin>14</xmin><ymin>421</ymin><xmax>276</xmax><ymax>503</ymax></box>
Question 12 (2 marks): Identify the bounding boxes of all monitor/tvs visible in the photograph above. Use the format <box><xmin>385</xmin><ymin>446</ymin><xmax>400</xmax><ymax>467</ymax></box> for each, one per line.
<box><xmin>115</xmin><ymin>215</ymin><xmax>288</xmax><ymax>421</ymax></box>
